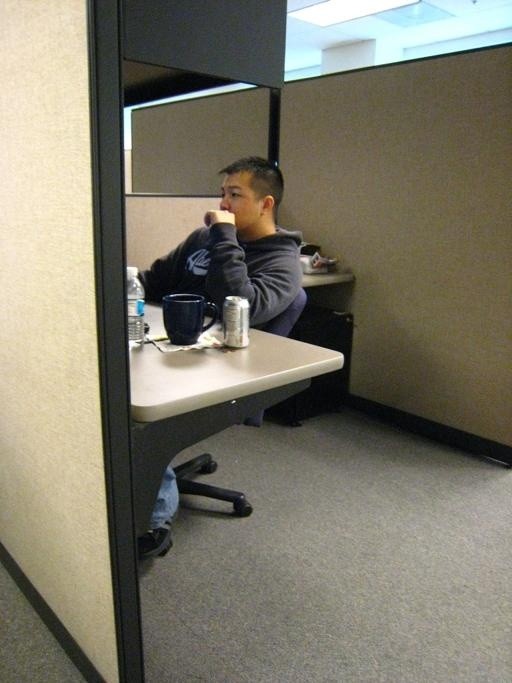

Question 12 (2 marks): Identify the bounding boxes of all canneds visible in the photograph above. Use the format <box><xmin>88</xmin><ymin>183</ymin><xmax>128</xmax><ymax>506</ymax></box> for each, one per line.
<box><xmin>222</xmin><ymin>295</ymin><xmax>250</xmax><ymax>348</ymax></box>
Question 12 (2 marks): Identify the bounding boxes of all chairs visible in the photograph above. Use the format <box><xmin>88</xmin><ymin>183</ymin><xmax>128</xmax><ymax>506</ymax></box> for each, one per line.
<box><xmin>173</xmin><ymin>286</ymin><xmax>336</xmax><ymax>516</ymax></box>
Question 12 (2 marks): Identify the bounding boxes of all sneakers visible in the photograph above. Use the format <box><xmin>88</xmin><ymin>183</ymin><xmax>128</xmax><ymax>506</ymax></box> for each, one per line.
<box><xmin>138</xmin><ymin>519</ymin><xmax>176</xmax><ymax>576</ymax></box>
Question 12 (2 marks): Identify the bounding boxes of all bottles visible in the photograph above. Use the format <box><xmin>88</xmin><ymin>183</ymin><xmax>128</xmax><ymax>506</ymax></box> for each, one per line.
<box><xmin>127</xmin><ymin>266</ymin><xmax>145</xmax><ymax>354</ymax></box>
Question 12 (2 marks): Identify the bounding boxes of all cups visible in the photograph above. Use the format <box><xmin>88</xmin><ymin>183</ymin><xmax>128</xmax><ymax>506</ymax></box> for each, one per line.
<box><xmin>161</xmin><ymin>293</ymin><xmax>220</xmax><ymax>346</ymax></box>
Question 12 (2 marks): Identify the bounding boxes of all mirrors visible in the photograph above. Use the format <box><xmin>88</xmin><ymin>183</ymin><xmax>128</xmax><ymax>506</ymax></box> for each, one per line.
<box><xmin>119</xmin><ymin>60</ymin><xmax>280</xmax><ymax>198</ymax></box>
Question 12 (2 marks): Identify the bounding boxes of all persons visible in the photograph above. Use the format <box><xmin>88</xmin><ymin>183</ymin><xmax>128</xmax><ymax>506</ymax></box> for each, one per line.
<box><xmin>133</xmin><ymin>153</ymin><xmax>305</xmax><ymax>564</ymax></box>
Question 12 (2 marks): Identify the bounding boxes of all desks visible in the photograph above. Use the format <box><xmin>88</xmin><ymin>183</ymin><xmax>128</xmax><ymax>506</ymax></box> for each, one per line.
<box><xmin>300</xmin><ymin>269</ymin><xmax>353</xmax><ymax>287</ymax></box>
<box><xmin>128</xmin><ymin>301</ymin><xmax>345</xmax><ymax>539</ymax></box>
